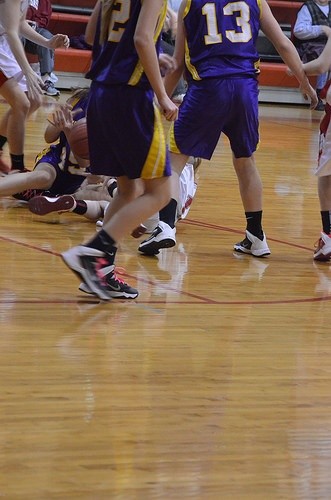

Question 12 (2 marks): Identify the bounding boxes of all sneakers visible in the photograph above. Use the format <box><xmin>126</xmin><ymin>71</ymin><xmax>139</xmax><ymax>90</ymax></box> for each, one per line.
<box><xmin>78</xmin><ymin>266</ymin><xmax>139</xmax><ymax>300</ymax></box>
<box><xmin>39</xmin><ymin>80</ymin><xmax>60</xmax><ymax>96</ymax></box>
<box><xmin>137</xmin><ymin>221</ymin><xmax>177</xmax><ymax>255</ymax></box>
<box><xmin>13</xmin><ymin>187</ymin><xmax>45</xmax><ymax>201</ymax></box>
<box><xmin>27</xmin><ymin>195</ymin><xmax>76</xmax><ymax>215</ymax></box>
<box><xmin>61</xmin><ymin>238</ymin><xmax>117</xmax><ymax>300</ymax></box>
<box><xmin>9</xmin><ymin>168</ymin><xmax>31</xmax><ymax>176</ymax></box>
<box><xmin>233</xmin><ymin>229</ymin><xmax>270</xmax><ymax>257</ymax></box>
<box><xmin>0</xmin><ymin>155</ymin><xmax>10</xmax><ymax>177</ymax></box>
<box><xmin>312</xmin><ymin>230</ymin><xmax>331</xmax><ymax>262</ymax></box>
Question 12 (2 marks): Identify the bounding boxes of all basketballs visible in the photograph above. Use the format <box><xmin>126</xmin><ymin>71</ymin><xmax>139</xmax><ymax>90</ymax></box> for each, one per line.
<box><xmin>69</xmin><ymin>121</ymin><xmax>93</xmax><ymax>159</ymax></box>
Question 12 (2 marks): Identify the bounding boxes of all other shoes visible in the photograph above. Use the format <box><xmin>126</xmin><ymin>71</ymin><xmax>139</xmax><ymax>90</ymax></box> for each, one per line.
<box><xmin>130</xmin><ymin>223</ymin><xmax>147</xmax><ymax>238</ymax></box>
<box><xmin>172</xmin><ymin>93</ymin><xmax>186</xmax><ymax>103</ymax></box>
<box><xmin>315</xmin><ymin>99</ymin><xmax>324</xmax><ymax>111</ymax></box>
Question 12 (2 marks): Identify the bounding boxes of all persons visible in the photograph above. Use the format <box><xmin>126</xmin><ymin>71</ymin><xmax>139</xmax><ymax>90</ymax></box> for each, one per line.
<box><xmin>290</xmin><ymin>0</ymin><xmax>331</xmax><ymax>110</ymax></box>
<box><xmin>0</xmin><ymin>0</ymin><xmax>70</xmax><ymax>177</ymax></box>
<box><xmin>22</xmin><ymin>0</ymin><xmax>60</xmax><ymax>95</ymax></box>
<box><xmin>154</xmin><ymin>0</ymin><xmax>317</xmax><ymax>256</ymax></box>
<box><xmin>61</xmin><ymin>0</ymin><xmax>179</xmax><ymax>301</ymax></box>
<box><xmin>0</xmin><ymin>86</ymin><xmax>203</xmax><ymax>254</ymax></box>
<box><xmin>286</xmin><ymin>29</ymin><xmax>331</xmax><ymax>262</ymax></box>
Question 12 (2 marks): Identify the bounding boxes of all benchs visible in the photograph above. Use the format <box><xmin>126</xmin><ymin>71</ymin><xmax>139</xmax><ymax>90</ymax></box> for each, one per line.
<box><xmin>25</xmin><ymin>0</ymin><xmax>317</xmax><ymax>104</ymax></box>
